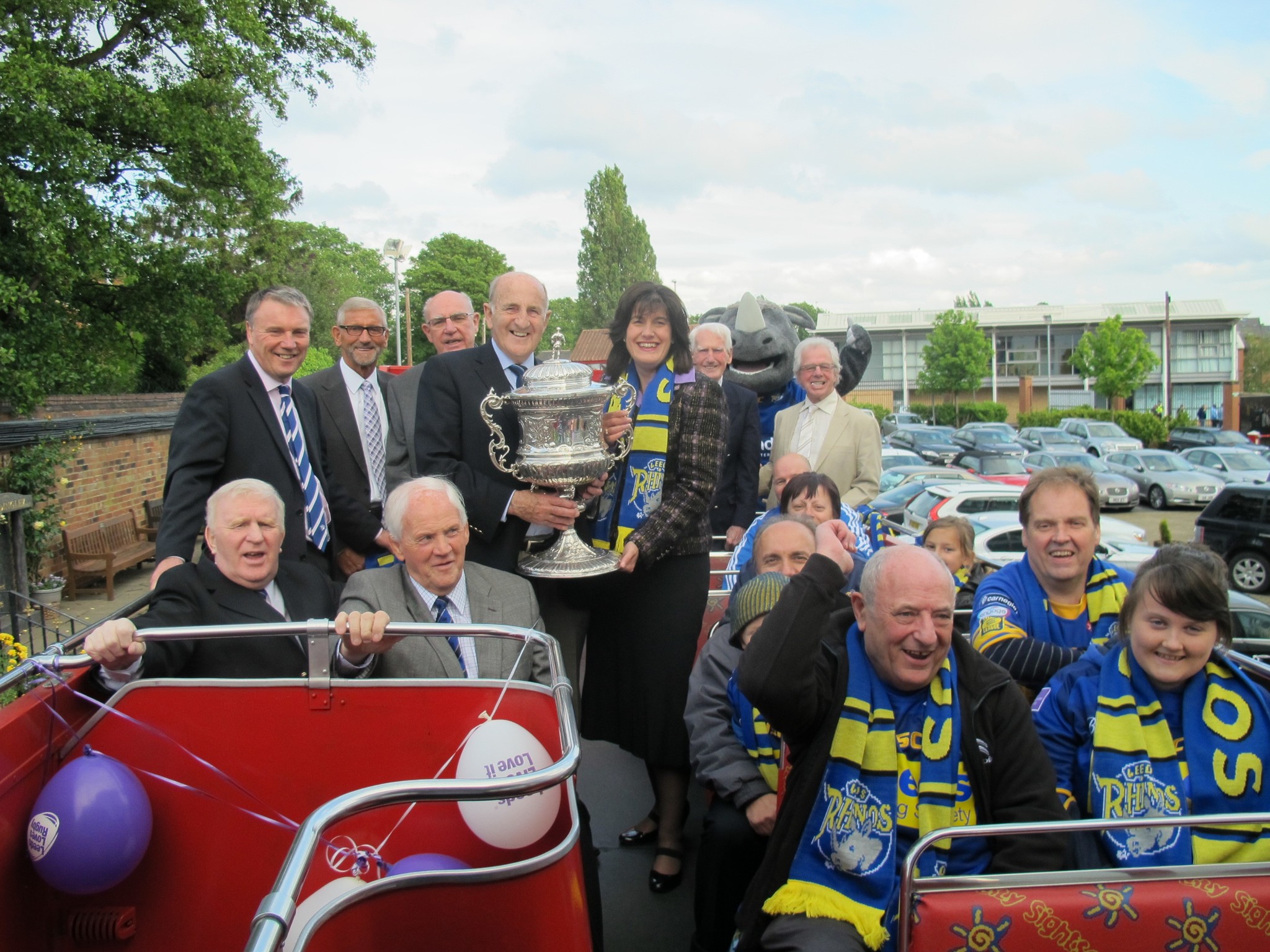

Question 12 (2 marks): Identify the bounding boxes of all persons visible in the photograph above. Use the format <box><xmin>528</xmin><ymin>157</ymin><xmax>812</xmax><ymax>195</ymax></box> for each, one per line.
<box><xmin>1210</xmin><ymin>405</ymin><xmax>1218</xmax><ymax>427</ymax></box>
<box><xmin>729</xmin><ymin>571</ymin><xmax>794</xmax><ymax>951</ymax></box>
<box><xmin>691</xmin><ymin>322</ymin><xmax>761</xmax><ymax>556</ymax></box>
<box><xmin>383</xmin><ymin>289</ymin><xmax>479</xmax><ymax>492</ymax></box>
<box><xmin>86</xmin><ymin>478</ymin><xmax>314</xmax><ymax>682</ymax></box>
<box><xmin>730</xmin><ymin>521</ymin><xmax>1081</xmax><ymax>952</ymax></box>
<box><xmin>923</xmin><ymin>516</ymin><xmax>986</xmax><ymax>633</ymax></box>
<box><xmin>1218</xmin><ymin>404</ymin><xmax>1224</xmax><ymax>427</ymax></box>
<box><xmin>296</xmin><ymin>297</ymin><xmax>395</xmax><ymax>572</ymax></box>
<box><xmin>329</xmin><ymin>472</ymin><xmax>560</xmax><ymax>690</ymax></box>
<box><xmin>685</xmin><ymin>518</ymin><xmax>817</xmax><ymax>952</ymax></box>
<box><xmin>971</xmin><ymin>462</ymin><xmax>1137</xmax><ymax>700</ymax></box>
<box><xmin>1198</xmin><ymin>405</ymin><xmax>1207</xmax><ymax>427</ymax></box>
<box><xmin>760</xmin><ymin>337</ymin><xmax>884</xmax><ymax>510</ymax></box>
<box><xmin>153</xmin><ymin>285</ymin><xmax>349</xmax><ymax>622</ymax></box>
<box><xmin>1177</xmin><ymin>404</ymin><xmax>1185</xmax><ymax>414</ymax></box>
<box><xmin>413</xmin><ymin>271</ymin><xmax>611</xmax><ymax>731</ymax></box>
<box><xmin>720</xmin><ymin>452</ymin><xmax>874</xmax><ymax>596</ymax></box>
<box><xmin>1030</xmin><ymin>542</ymin><xmax>1269</xmax><ymax>866</ymax></box>
<box><xmin>726</xmin><ymin>472</ymin><xmax>866</xmax><ymax>618</ymax></box>
<box><xmin>1152</xmin><ymin>401</ymin><xmax>1163</xmax><ymax>418</ymax></box>
<box><xmin>579</xmin><ymin>283</ymin><xmax>729</xmax><ymax>891</ymax></box>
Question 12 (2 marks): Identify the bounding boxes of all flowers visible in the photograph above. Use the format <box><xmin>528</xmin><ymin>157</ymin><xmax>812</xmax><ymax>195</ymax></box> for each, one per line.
<box><xmin>30</xmin><ymin>574</ymin><xmax>66</xmax><ymax>591</ymax></box>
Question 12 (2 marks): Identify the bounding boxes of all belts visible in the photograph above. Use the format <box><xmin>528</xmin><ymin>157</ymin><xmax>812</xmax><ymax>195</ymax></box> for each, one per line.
<box><xmin>369</xmin><ymin>507</ymin><xmax>383</xmax><ymax>519</ymax></box>
<box><xmin>521</xmin><ymin>537</ymin><xmax>554</xmax><ymax>554</ymax></box>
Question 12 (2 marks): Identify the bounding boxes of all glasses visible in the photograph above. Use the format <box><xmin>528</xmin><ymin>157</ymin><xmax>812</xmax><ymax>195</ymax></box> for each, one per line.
<box><xmin>798</xmin><ymin>362</ymin><xmax>835</xmax><ymax>374</ymax></box>
<box><xmin>427</xmin><ymin>313</ymin><xmax>473</xmax><ymax>331</ymax></box>
<box><xmin>339</xmin><ymin>325</ymin><xmax>386</xmax><ymax>337</ymax></box>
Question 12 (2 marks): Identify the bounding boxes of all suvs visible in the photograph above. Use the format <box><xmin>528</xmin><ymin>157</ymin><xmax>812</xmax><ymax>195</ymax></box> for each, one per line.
<box><xmin>1167</xmin><ymin>427</ymin><xmax>1270</xmax><ymax>460</ymax></box>
<box><xmin>1058</xmin><ymin>418</ymin><xmax>1142</xmax><ymax>458</ymax></box>
<box><xmin>900</xmin><ymin>482</ymin><xmax>1147</xmax><ymax>545</ymax></box>
<box><xmin>1187</xmin><ymin>479</ymin><xmax>1270</xmax><ymax>595</ymax></box>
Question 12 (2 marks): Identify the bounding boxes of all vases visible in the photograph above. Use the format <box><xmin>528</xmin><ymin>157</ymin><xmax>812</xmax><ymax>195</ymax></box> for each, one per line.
<box><xmin>32</xmin><ymin>585</ymin><xmax>65</xmax><ymax>604</ymax></box>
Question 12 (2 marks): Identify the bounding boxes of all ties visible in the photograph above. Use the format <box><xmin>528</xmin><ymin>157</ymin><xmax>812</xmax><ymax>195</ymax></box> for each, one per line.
<box><xmin>278</xmin><ymin>384</ymin><xmax>328</xmax><ymax>553</ymax></box>
<box><xmin>509</xmin><ymin>364</ymin><xmax>528</xmax><ymax>389</ymax></box>
<box><xmin>360</xmin><ymin>380</ymin><xmax>387</xmax><ymax>509</ymax></box>
<box><xmin>797</xmin><ymin>405</ymin><xmax>820</xmax><ymax>459</ymax></box>
<box><xmin>434</xmin><ymin>595</ymin><xmax>469</xmax><ymax>679</ymax></box>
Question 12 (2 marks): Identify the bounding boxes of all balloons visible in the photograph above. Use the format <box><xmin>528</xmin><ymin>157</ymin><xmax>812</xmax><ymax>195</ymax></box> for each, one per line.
<box><xmin>28</xmin><ymin>743</ymin><xmax>154</xmax><ymax>896</ymax></box>
<box><xmin>454</xmin><ymin>710</ymin><xmax>563</xmax><ymax>853</ymax></box>
<box><xmin>387</xmin><ymin>853</ymin><xmax>475</xmax><ymax>880</ymax></box>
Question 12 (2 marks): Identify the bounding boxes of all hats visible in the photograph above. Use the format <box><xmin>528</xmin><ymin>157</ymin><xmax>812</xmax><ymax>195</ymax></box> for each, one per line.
<box><xmin>729</xmin><ymin>572</ymin><xmax>790</xmax><ymax>651</ymax></box>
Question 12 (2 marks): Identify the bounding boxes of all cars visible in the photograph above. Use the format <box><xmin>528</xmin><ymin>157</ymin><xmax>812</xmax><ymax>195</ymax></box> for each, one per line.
<box><xmin>1222</xmin><ymin>587</ymin><xmax>1270</xmax><ymax>665</ymax></box>
<box><xmin>966</xmin><ymin>515</ymin><xmax>1161</xmax><ymax>577</ymax></box>
<box><xmin>1176</xmin><ymin>445</ymin><xmax>1270</xmax><ymax>484</ymax></box>
<box><xmin>1012</xmin><ymin>427</ymin><xmax>1087</xmax><ymax>453</ymax></box>
<box><xmin>1021</xmin><ymin>451</ymin><xmax>1140</xmax><ymax>513</ymax></box>
<box><xmin>861</xmin><ymin>409</ymin><xmax>1034</xmax><ymax>535</ymax></box>
<box><xmin>1098</xmin><ymin>448</ymin><xmax>1226</xmax><ymax>511</ymax></box>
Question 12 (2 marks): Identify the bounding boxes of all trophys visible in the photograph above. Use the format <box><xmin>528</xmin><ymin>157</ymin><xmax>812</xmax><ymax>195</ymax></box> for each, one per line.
<box><xmin>480</xmin><ymin>326</ymin><xmax>624</xmax><ymax>580</ymax></box>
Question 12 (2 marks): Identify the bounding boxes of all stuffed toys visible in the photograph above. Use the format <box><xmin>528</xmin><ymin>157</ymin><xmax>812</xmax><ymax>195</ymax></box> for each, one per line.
<box><xmin>696</xmin><ymin>293</ymin><xmax>873</xmax><ymax>467</ymax></box>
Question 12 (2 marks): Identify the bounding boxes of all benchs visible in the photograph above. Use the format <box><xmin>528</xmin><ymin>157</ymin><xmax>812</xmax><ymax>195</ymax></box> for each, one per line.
<box><xmin>62</xmin><ymin>508</ymin><xmax>157</xmax><ymax>600</ymax></box>
<box><xmin>144</xmin><ymin>498</ymin><xmax>166</xmax><ymax>542</ymax></box>
<box><xmin>697</xmin><ymin>537</ymin><xmax>1269</xmax><ymax>952</ymax></box>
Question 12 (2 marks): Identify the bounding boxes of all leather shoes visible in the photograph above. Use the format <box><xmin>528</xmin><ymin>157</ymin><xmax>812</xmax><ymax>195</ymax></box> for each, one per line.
<box><xmin>619</xmin><ymin>798</ymin><xmax>690</xmax><ymax>849</ymax></box>
<box><xmin>648</xmin><ymin>847</ymin><xmax>688</xmax><ymax>895</ymax></box>
<box><xmin>729</xmin><ymin>927</ymin><xmax>761</xmax><ymax>952</ymax></box>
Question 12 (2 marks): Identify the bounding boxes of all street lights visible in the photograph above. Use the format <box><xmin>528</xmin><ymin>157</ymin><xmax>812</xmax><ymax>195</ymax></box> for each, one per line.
<box><xmin>383</xmin><ymin>238</ymin><xmax>413</xmax><ymax>366</ymax></box>
